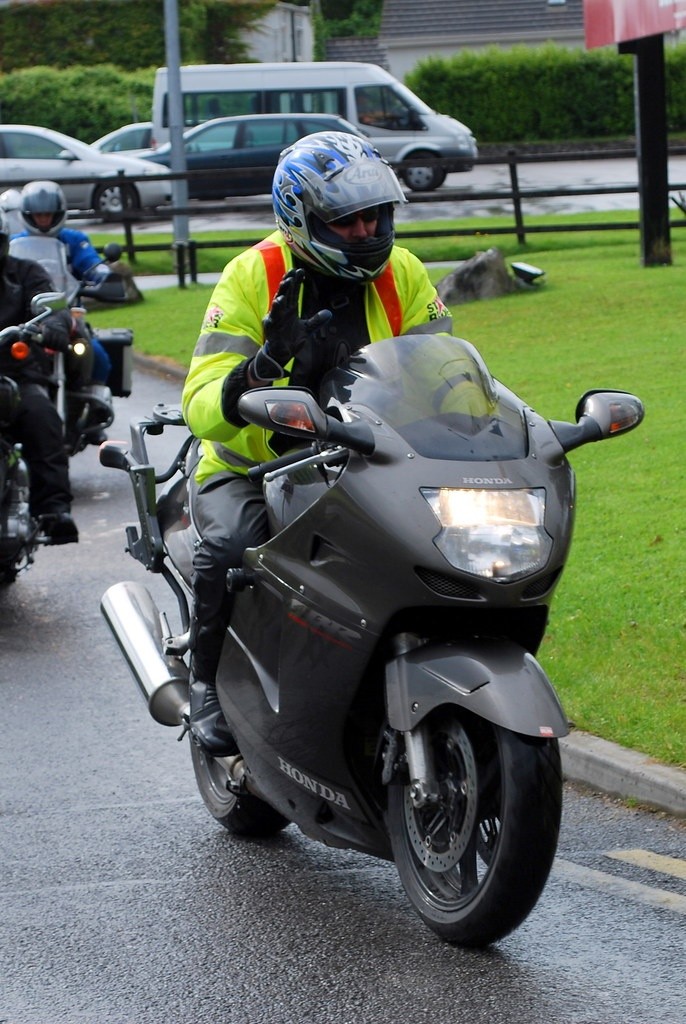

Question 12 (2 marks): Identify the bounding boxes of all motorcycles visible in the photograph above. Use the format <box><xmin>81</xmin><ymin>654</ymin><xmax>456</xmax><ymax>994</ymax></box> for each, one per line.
<box><xmin>100</xmin><ymin>334</ymin><xmax>645</xmax><ymax>948</ymax></box>
<box><xmin>0</xmin><ymin>238</ymin><xmax>133</xmax><ymax>584</ymax></box>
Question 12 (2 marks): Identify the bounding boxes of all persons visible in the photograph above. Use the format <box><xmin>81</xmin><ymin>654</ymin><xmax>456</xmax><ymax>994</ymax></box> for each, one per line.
<box><xmin>0</xmin><ymin>180</ymin><xmax>110</xmax><ymax>541</ymax></box>
<box><xmin>182</xmin><ymin>132</ymin><xmax>501</xmax><ymax>755</ymax></box>
<box><xmin>357</xmin><ymin>90</ymin><xmax>396</xmax><ymax>124</ymax></box>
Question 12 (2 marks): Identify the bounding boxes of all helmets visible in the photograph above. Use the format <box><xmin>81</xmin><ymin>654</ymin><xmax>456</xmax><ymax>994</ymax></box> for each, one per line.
<box><xmin>272</xmin><ymin>131</ymin><xmax>396</xmax><ymax>281</ymax></box>
<box><xmin>21</xmin><ymin>181</ymin><xmax>66</xmax><ymax>236</ymax></box>
<box><xmin>0</xmin><ymin>207</ymin><xmax>10</xmax><ymax>260</ymax></box>
<box><xmin>0</xmin><ymin>190</ymin><xmax>22</xmax><ymax>213</ymax></box>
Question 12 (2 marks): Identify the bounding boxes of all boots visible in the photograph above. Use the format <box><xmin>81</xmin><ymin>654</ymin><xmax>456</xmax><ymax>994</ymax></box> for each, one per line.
<box><xmin>188</xmin><ymin>572</ymin><xmax>241</xmax><ymax>757</ymax></box>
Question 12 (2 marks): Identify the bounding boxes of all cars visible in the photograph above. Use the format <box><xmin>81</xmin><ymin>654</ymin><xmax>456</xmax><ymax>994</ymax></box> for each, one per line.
<box><xmin>138</xmin><ymin>113</ymin><xmax>377</xmax><ymax>198</ymax></box>
<box><xmin>0</xmin><ymin>121</ymin><xmax>172</xmax><ymax>221</ymax></box>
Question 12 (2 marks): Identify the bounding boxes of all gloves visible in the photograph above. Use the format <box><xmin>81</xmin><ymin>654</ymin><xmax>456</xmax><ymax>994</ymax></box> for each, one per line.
<box><xmin>40</xmin><ymin>315</ymin><xmax>70</xmax><ymax>350</ymax></box>
<box><xmin>249</xmin><ymin>268</ymin><xmax>332</xmax><ymax>383</ymax></box>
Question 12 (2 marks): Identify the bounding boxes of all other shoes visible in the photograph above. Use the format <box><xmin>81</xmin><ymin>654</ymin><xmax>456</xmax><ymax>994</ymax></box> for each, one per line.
<box><xmin>36</xmin><ymin>513</ymin><xmax>78</xmax><ymax>536</ymax></box>
<box><xmin>90</xmin><ymin>430</ymin><xmax>107</xmax><ymax>445</ymax></box>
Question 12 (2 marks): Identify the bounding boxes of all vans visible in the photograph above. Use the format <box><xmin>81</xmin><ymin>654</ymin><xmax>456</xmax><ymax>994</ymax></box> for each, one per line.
<box><xmin>151</xmin><ymin>63</ymin><xmax>479</xmax><ymax>201</ymax></box>
